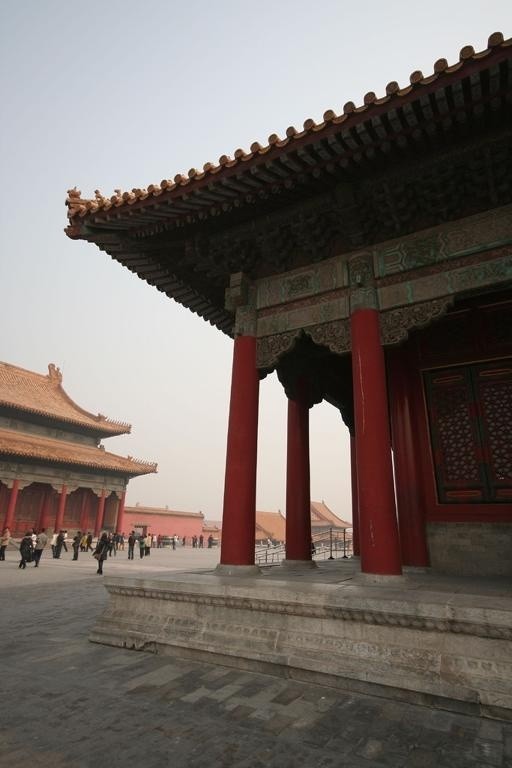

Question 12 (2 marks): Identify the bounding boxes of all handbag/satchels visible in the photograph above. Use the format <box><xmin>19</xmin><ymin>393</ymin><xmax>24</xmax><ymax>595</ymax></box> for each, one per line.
<box><xmin>27</xmin><ymin>548</ymin><xmax>34</xmax><ymax>561</ymax></box>
<box><xmin>94</xmin><ymin>553</ymin><xmax>101</xmax><ymax>560</ymax></box>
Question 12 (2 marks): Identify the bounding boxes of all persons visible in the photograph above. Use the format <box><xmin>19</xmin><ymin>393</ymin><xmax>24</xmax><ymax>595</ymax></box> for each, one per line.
<box><xmin>96</xmin><ymin>532</ymin><xmax>109</xmax><ymax>574</ymax></box>
<box><xmin>108</xmin><ymin>530</ymin><xmax>168</xmax><ymax>560</ymax></box>
<box><xmin>172</xmin><ymin>533</ymin><xmax>213</xmax><ymax>551</ymax></box>
<box><xmin>0</xmin><ymin>526</ymin><xmax>94</xmax><ymax>569</ymax></box>
